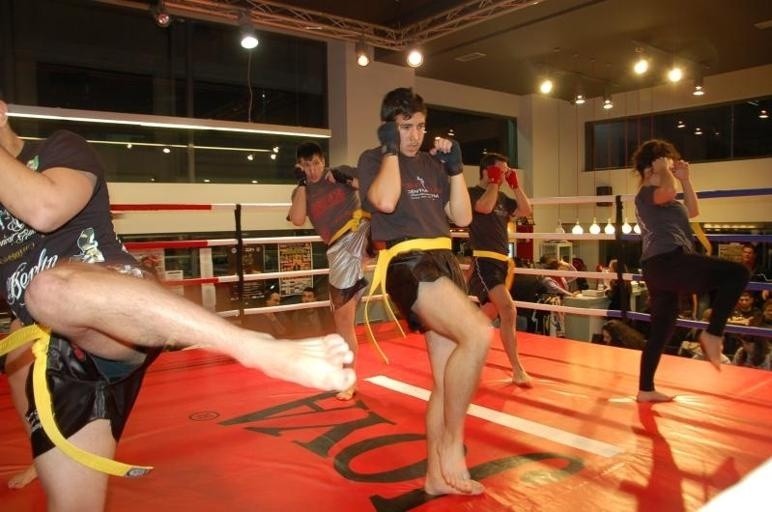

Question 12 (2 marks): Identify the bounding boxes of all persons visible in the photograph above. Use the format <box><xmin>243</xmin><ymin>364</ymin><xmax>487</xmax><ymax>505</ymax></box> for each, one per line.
<box><xmin>0</xmin><ymin>99</ymin><xmax>357</xmax><ymax>512</ymax></box>
<box><xmin>292</xmin><ymin>287</ymin><xmax>336</xmax><ymax>336</ymax></box>
<box><xmin>286</xmin><ymin>141</ymin><xmax>379</xmax><ymax>400</ymax></box>
<box><xmin>509</xmin><ymin>245</ymin><xmax>771</xmax><ymax>371</ymax></box>
<box><xmin>230</xmin><ymin>255</ymin><xmax>265</xmax><ymax>299</ymax></box>
<box><xmin>357</xmin><ymin>85</ymin><xmax>486</xmax><ymax>498</ymax></box>
<box><xmin>470</xmin><ymin>153</ymin><xmax>532</xmax><ymax>386</ymax></box>
<box><xmin>247</xmin><ymin>289</ymin><xmax>303</xmax><ymax>339</ymax></box>
<box><xmin>635</xmin><ymin>140</ymin><xmax>749</xmax><ymax>401</ymax></box>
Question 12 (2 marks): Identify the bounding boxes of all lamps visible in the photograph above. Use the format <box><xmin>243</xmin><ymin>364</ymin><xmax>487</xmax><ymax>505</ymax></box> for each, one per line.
<box><xmin>117</xmin><ymin>139</ymin><xmax>283</xmax><ymax>164</ymax></box>
<box><xmin>757</xmin><ymin>104</ymin><xmax>770</xmax><ymax>121</ymax></box>
<box><xmin>146</xmin><ymin>2</ymin><xmax>428</xmax><ymax>77</ymax></box>
<box><xmin>676</xmin><ymin>117</ymin><xmax>722</xmax><ymax>139</ymax></box>
<box><xmin>632</xmin><ymin>37</ymin><xmax>715</xmax><ymax>97</ymax></box>
<box><xmin>533</xmin><ymin>60</ymin><xmax>621</xmax><ymax>111</ymax></box>
<box><xmin>554</xmin><ymin>64</ymin><xmax>642</xmax><ymax>238</ymax></box>
